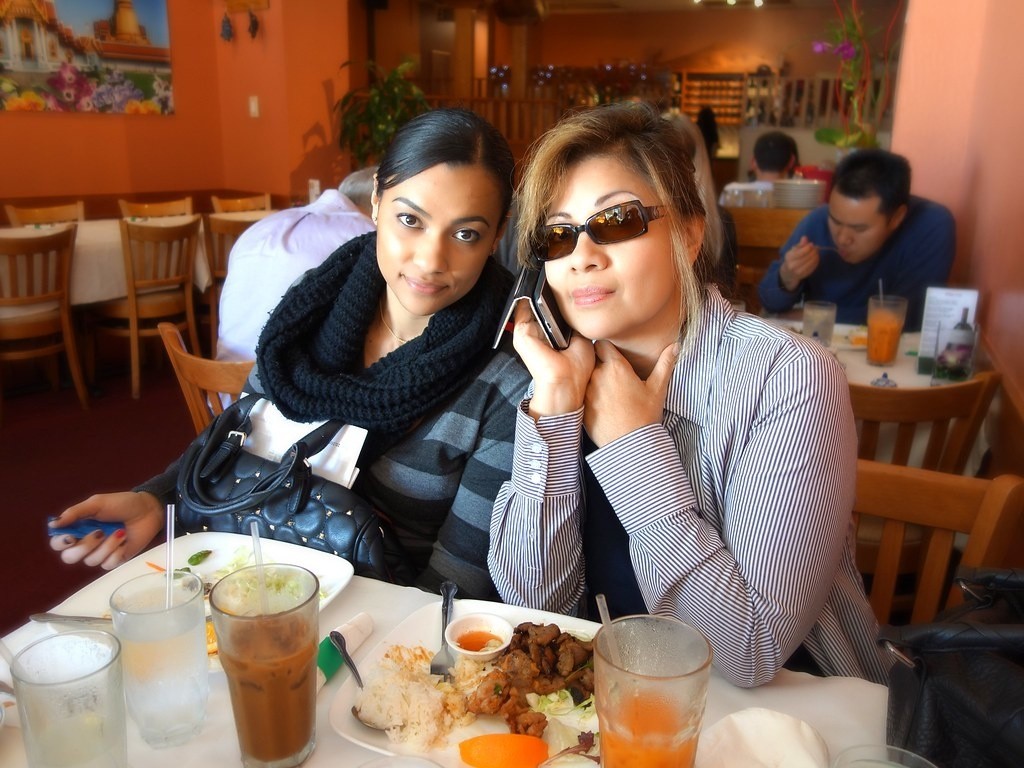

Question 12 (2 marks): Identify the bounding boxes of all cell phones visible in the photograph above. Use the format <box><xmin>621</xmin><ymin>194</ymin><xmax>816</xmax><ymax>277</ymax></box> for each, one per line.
<box><xmin>534</xmin><ymin>260</ymin><xmax>573</xmax><ymax>349</ymax></box>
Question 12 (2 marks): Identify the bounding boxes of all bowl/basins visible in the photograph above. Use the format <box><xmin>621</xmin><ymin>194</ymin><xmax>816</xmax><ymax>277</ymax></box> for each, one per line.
<box><xmin>443</xmin><ymin>613</ymin><xmax>513</xmax><ymax>663</ymax></box>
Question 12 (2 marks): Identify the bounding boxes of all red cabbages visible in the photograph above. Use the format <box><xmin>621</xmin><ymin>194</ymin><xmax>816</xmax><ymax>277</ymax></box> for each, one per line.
<box><xmin>165</xmin><ymin>549</ymin><xmax>328</xmax><ymax>606</ymax></box>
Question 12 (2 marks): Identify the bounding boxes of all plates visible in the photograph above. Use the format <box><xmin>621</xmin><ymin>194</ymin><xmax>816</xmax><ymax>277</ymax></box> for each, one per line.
<box><xmin>792</xmin><ymin>322</ymin><xmax>872</xmax><ymax>351</ymax></box>
<box><xmin>1</xmin><ymin>531</ymin><xmax>354</xmax><ymax>727</ymax></box>
<box><xmin>330</xmin><ymin>594</ymin><xmax>601</xmax><ymax>768</ymax></box>
<box><xmin>722</xmin><ymin>177</ymin><xmax>827</xmax><ymax>207</ymax></box>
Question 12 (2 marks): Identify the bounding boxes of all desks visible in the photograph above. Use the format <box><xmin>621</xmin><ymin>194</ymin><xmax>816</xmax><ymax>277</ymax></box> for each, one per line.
<box><xmin>1</xmin><ymin>211</ymin><xmax>278</xmax><ymax>324</ymax></box>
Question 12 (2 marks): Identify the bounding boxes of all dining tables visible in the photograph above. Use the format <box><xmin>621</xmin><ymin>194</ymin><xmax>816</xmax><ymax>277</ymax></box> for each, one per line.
<box><xmin>0</xmin><ymin>575</ymin><xmax>899</xmax><ymax>768</ymax></box>
<box><xmin>781</xmin><ymin>324</ymin><xmax>1000</xmax><ymax>481</ymax></box>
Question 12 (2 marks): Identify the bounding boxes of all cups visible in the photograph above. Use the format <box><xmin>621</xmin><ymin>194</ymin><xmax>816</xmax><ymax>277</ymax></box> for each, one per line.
<box><xmin>592</xmin><ymin>614</ymin><xmax>713</xmax><ymax>767</ymax></box>
<box><xmin>803</xmin><ymin>301</ymin><xmax>838</xmax><ymax>348</ymax></box>
<box><xmin>111</xmin><ymin>569</ymin><xmax>208</xmax><ymax>750</ymax></box>
<box><xmin>866</xmin><ymin>294</ymin><xmax>908</xmax><ymax>367</ymax></box>
<box><xmin>9</xmin><ymin>628</ymin><xmax>128</xmax><ymax>766</ymax></box>
<box><xmin>831</xmin><ymin>741</ymin><xmax>936</xmax><ymax>767</ymax></box>
<box><xmin>207</xmin><ymin>565</ymin><xmax>319</xmax><ymax>767</ymax></box>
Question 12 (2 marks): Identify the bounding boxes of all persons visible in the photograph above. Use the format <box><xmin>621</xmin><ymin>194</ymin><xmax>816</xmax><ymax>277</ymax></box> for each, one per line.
<box><xmin>657</xmin><ymin>110</ymin><xmax>741</xmax><ymax>300</ymax></box>
<box><xmin>46</xmin><ymin>107</ymin><xmax>535</xmax><ymax>603</ymax></box>
<box><xmin>696</xmin><ymin>105</ymin><xmax>736</xmax><ymax>194</ymax></box>
<box><xmin>718</xmin><ymin>131</ymin><xmax>800</xmax><ymax>210</ymax></box>
<box><xmin>755</xmin><ymin>147</ymin><xmax>958</xmax><ymax>334</ymax></box>
<box><xmin>483</xmin><ymin>101</ymin><xmax>913</xmax><ymax>691</ymax></box>
<box><xmin>208</xmin><ymin>162</ymin><xmax>379</xmax><ymax>416</ymax></box>
<box><xmin>746</xmin><ymin>64</ymin><xmax>789</xmax><ymax>127</ymax></box>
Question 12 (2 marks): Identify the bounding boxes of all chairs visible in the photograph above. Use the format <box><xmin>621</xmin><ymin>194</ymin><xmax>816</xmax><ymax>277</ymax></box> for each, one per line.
<box><xmin>846</xmin><ymin>366</ymin><xmax>1004</xmax><ymax>617</ymax></box>
<box><xmin>84</xmin><ymin>215</ymin><xmax>203</xmax><ymax>402</ymax></box>
<box><xmin>5</xmin><ymin>201</ymin><xmax>85</xmax><ymax>233</ymax></box>
<box><xmin>117</xmin><ymin>198</ymin><xmax>193</xmax><ymax>219</ymax></box>
<box><xmin>208</xmin><ymin>193</ymin><xmax>273</xmax><ymax>215</ymax></box>
<box><xmin>847</xmin><ymin>458</ymin><xmax>1024</xmax><ymax>628</ymax></box>
<box><xmin>190</xmin><ymin>212</ymin><xmax>265</xmax><ymax>362</ymax></box>
<box><xmin>1</xmin><ymin>222</ymin><xmax>90</xmax><ymax>412</ymax></box>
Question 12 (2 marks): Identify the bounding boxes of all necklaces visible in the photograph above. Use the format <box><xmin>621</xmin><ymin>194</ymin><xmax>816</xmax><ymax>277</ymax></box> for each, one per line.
<box><xmin>377</xmin><ymin>305</ymin><xmax>408</xmax><ymax>346</ymax></box>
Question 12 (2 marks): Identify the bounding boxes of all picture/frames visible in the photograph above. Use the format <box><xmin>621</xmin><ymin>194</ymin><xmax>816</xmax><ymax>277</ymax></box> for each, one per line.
<box><xmin>0</xmin><ymin>0</ymin><xmax>176</xmax><ymax>117</ymax></box>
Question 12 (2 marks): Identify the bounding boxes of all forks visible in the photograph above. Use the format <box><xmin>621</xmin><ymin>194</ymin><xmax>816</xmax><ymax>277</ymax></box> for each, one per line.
<box><xmin>430</xmin><ymin>581</ymin><xmax>457</xmax><ymax>685</ymax></box>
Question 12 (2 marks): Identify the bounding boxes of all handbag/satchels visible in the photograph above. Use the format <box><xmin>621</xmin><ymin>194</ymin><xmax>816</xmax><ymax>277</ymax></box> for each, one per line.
<box><xmin>177</xmin><ymin>392</ymin><xmax>414</xmax><ymax>586</ymax></box>
<box><xmin>878</xmin><ymin>570</ymin><xmax>1023</xmax><ymax>768</ymax></box>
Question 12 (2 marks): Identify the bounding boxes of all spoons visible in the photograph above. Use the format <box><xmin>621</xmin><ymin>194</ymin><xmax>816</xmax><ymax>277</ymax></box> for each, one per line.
<box><xmin>329</xmin><ymin>629</ymin><xmax>390</xmax><ymax>732</ymax></box>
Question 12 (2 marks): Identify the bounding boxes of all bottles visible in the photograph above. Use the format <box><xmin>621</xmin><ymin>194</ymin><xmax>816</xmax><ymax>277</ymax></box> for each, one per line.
<box><xmin>945</xmin><ymin>306</ymin><xmax>974</xmax><ymax>367</ymax></box>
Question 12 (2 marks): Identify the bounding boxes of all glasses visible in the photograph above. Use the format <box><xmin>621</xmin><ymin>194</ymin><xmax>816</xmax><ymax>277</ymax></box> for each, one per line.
<box><xmin>530</xmin><ymin>198</ymin><xmax>673</xmax><ymax>262</ymax></box>
